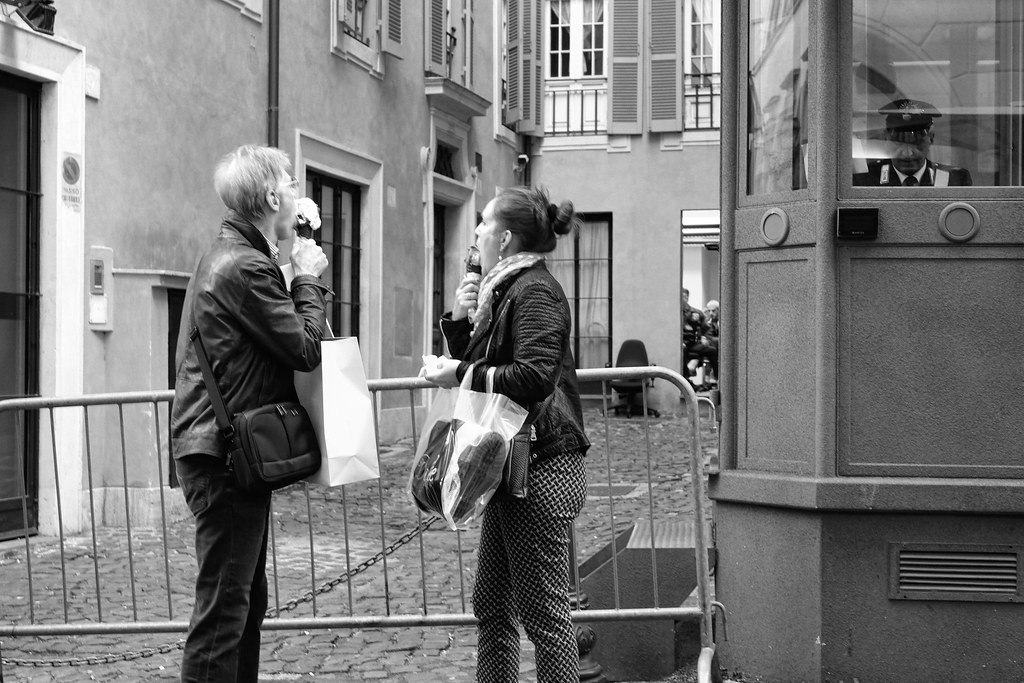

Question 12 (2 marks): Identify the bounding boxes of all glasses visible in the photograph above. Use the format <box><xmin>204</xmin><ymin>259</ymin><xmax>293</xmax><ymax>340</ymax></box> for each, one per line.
<box><xmin>277</xmin><ymin>177</ymin><xmax>300</xmax><ymax>189</ymax></box>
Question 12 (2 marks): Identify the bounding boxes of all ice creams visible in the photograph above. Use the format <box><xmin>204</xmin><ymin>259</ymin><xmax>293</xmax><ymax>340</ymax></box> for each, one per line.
<box><xmin>465</xmin><ymin>245</ymin><xmax>482</xmax><ymax>280</ymax></box>
<box><xmin>296</xmin><ymin>197</ymin><xmax>322</xmax><ymax>239</ymax></box>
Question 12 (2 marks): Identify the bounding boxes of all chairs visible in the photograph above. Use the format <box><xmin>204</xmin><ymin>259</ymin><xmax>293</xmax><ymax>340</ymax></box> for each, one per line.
<box><xmin>598</xmin><ymin>339</ymin><xmax>662</xmax><ymax>420</ymax></box>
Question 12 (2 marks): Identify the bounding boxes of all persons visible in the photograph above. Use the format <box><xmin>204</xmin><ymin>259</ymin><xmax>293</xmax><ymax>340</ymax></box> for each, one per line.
<box><xmin>682</xmin><ymin>289</ymin><xmax>720</xmax><ymax>392</ymax></box>
<box><xmin>423</xmin><ymin>186</ymin><xmax>589</xmax><ymax>681</ymax></box>
<box><xmin>852</xmin><ymin>98</ymin><xmax>975</xmax><ymax>186</ymax></box>
<box><xmin>700</xmin><ymin>300</ymin><xmax>720</xmax><ymax>347</ymax></box>
<box><xmin>171</xmin><ymin>143</ymin><xmax>329</xmax><ymax>683</ymax></box>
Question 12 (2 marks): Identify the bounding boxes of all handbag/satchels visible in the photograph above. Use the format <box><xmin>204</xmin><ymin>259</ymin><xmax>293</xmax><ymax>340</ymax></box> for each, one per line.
<box><xmin>294</xmin><ymin>317</ymin><xmax>381</xmax><ymax>487</ymax></box>
<box><xmin>490</xmin><ymin>423</ymin><xmax>530</xmax><ymax>499</ymax></box>
<box><xmin>407</xmin><ymin>360</ymin><xmax>528</xmax><ymax>530</ymax></box>
<box><xmin>232</xmin><ymin>401</ymin><xmax>322</xmax><ymax>496</ymax></box>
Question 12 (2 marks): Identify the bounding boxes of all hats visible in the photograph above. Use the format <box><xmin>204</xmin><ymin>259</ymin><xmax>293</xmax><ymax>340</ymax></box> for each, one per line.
<box><xmin>879</xmin><ymin>99</ymin><xmax>941</xmax><ymax>130</ymax></box>
